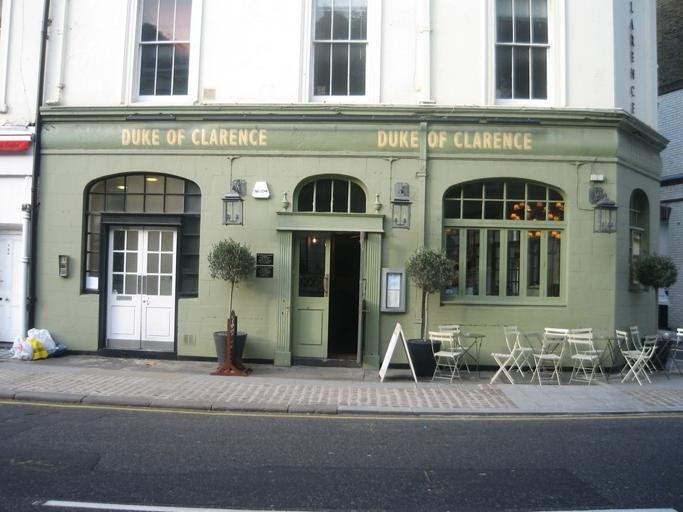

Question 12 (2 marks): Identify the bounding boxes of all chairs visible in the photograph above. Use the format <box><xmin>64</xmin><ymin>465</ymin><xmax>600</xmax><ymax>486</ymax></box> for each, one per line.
<box><xmin>427</xmin><ymin>325</ymin><xmax>683</xmax><ymax>386</ymax></box>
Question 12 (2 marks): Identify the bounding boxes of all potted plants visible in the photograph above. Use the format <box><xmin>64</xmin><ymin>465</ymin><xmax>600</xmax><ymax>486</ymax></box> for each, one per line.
<box><xmin>634</xmin><ymin>252</ymin><xmax>678</xmax><ymax>370</ymax></box>
<box><xmin>207</xmin><ymin>237</ymin><xmax>256</xmax><ymax>366</ymax></box>
<box><xmin>406</xmin><ymin>251</ymin><xmax>460</xmax><ymax>377</ymax></box>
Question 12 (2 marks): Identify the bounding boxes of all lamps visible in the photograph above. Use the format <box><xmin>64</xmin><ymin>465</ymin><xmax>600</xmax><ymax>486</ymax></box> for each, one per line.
<box><xmin>390</xmin><ymin>182</ymin><xmax>413</xmax><ymax>230</ymax></box>
<box><xmin>221</xmin><ymin>179</ymin><xmax>247</xmax><ymax>226</ymax></box>
<box><xmin>511</xmin><ymin>202</ymin><xmax>564</xmax><ymax>240</ymax></box>
<box><xmin>588</xmin><ymin>187</ymin><xmax>619</xmax><ymax>233</ymax></box>
